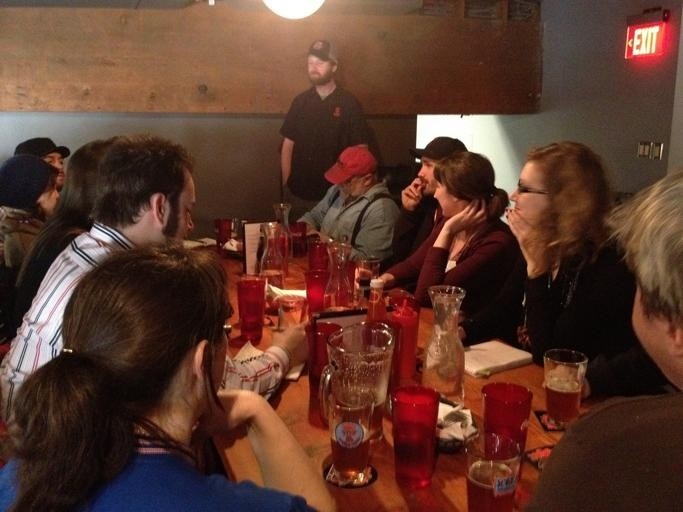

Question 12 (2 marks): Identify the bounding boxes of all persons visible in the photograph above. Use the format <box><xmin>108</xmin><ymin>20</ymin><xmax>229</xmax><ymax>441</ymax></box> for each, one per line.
<box><xmin>295</xmin><ymin>143</ymin><xmax>401</xmax><ymax>269</ymax></box>
<box><xmin>13</xmin><ymin>136</ymin><xmax>71</xmax><ymax>190</ymax></box>
<box><xmin>0</xmin><ymin>153</ymin><xmax>59</xmax><ymax>315</ymax></box>
<box><xmin>518</xmin><ymin>172</ymin><xmax>682</xmax><ymax>511</ymax></box>
<box><xmin>0</xmin><ymin>137</ymin><xmax>116</xmax><ymax>362</ymax></box>
<box><xmin>353</xmin><ymin>151</ymin><xmax>528</xmax><ymax>348</ymax></box>
<box><xmin>279</xmin><ymin>40</ymin><xmax>373</xmax><ymax>226</ymax></box>
<box><xmin>1</xmin><ymin>134</ymin><xmax>311</xmax><ymax>450</ymax></box>
<box><xmin>1</xmin><ymin>243</ymin><xmax>337</xmax><ymax>512</ymax></box>
<box><xmin>392</xmin><ymin>137</ymin><xmax>468</xmax><ymax>293</ymax></box>
<box><xmin>418</xmin><ymin>140</ymin><xmax>675</xmax><ymax>402</ymax></box>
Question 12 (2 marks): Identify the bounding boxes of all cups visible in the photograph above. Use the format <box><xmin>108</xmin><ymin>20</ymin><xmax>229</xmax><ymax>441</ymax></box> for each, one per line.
<box><xmin>331</xmin><ymin>388</ymin><xmax>374</xmax><ymax>485</ymax></box>
<box><xmin>390</xmin><ymin>384</ymin><xmax>439</xmax><ymax>479</ymax></box>
<box><xmin>209</xmin><ymin>201</ymin><xmax>481</xmax><ymax>455</ymax></box>
<box><xmin>466</xmin><ymin>431</ymin><xmax>520</xmax><ymax>512</ymax></box>
<box><xmin>479</xmin><ymin>382</ymin><xmax>534</xmax><ymax>466</ymax></box>
<box><xmin>541</xmin><ymin>348</ymin><xmax>589</xmax><ymax>424</ymax></box>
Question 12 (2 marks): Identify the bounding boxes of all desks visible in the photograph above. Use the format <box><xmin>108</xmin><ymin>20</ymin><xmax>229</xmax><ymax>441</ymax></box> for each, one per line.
<box><xmin>183</xmin><ymin>243</ymin><xmax>627</xmax><ymax>512</ymax></box>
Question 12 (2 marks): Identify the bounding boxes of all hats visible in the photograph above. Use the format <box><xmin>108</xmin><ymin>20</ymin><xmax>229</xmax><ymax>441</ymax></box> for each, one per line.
<box><xmin>410</xmin><ymin>137</ymin><xmax>467</xmax><ymax>160</ymax></box>
<box><xmin>324</xmin><ymin>145</ymin><xmax>377</xmax><ymax>183</ymax></box>
<box><xmin>310</xmin><ymin>40</ymin><xmax>336</xmax><ymax>60</ymax></box>
<box><xmin>15</xmin><ymin>137</ymin><xmax>70</xmax><ymax>160</ymax></box>
